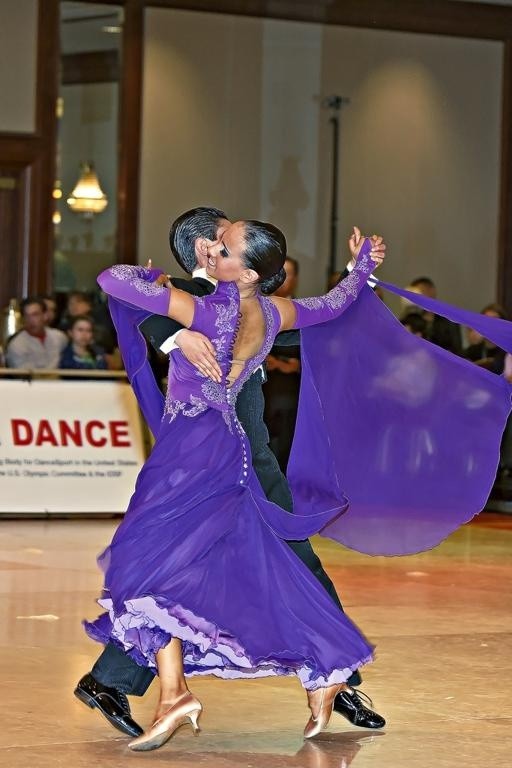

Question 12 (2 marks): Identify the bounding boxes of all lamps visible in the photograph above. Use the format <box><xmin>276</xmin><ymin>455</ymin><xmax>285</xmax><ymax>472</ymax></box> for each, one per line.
<box><xmin>67</xmin><ymin>159</ymin><xmax>108</xmax><ymax>214</ymax></box>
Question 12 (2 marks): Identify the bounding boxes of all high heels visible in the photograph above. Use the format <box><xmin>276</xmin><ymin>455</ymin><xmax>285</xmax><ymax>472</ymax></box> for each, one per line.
<box><xmin>127</xmin><ymin>691</ymin><xmax>203</xmax><ymax>751</ymax></box>
<box><xmin>303</xmin><ymin>682</ymin><xmax>355</xmax><ymax>738</ymax></box>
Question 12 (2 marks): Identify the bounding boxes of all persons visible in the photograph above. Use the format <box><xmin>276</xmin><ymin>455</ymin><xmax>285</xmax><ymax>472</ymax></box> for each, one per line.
<box><xmin>1</xmin><ymin>288</ymin><xmax>169</xmax><ymax>390</ymax></box>
<box><xmin>260</xmin><ymin>255</ymin><xmax>302</xmax><ymax>480</ymax></box>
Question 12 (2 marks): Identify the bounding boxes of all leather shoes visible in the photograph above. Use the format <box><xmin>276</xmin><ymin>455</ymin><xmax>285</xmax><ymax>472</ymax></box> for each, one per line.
<box><xmin>333</xmin><ymin>687</ymin><xmax>386</xmax><ymax>729</ymax></box>
<box><xmin>74</xmin><ymin>671</ymin><xmax>144</xmax><ymax>738</ymax></box>
